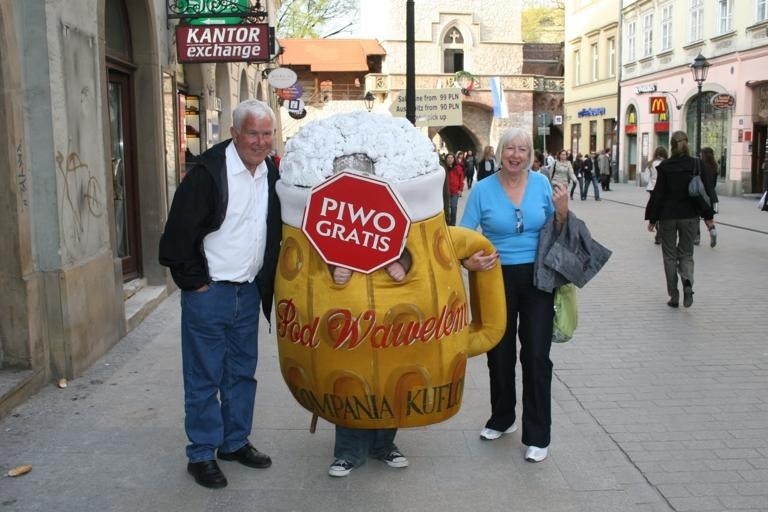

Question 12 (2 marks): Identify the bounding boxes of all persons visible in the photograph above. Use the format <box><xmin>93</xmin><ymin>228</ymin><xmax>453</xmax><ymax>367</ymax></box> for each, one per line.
<box><xmin>644</xmin><ymin>130</ymin><xmax>717</xmax><ymax>307</ymax></box>
<box><xmin>158</xmin><ymin>99</ymin><xmax>280</xmax><ymax>489</ymax></box>
<box><xmin>327</xmin><ymin>247</ymin><xmax>412</xmax><ymax>477</ymax></box>
<box><xmin>693</xmin><ymin>147</ymin><xmax>718</xmax><ymax>247</ymax></box>
<box><xmin>429</xmin><ymin>128</ymin><xmax>620</xmax><ymax>202</ymax></box>
<box><xmin>638</xmin><ymin>147</ymin><xmax>668</xmax><ymax>195</ymax></box>
<box><xmin>717</xmin><ymin>148</ymin><xmax>726</xmax><ymax>180</ymax></box>
<box><xmin>458</xmin><ymin>128</ymin><xmax>568</xmax><ymax>462</ymax></box>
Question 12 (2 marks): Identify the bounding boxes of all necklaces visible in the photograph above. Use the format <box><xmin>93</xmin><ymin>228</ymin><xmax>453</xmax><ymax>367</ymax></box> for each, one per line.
<box><xmin>505</xmin><ymin>175</ymin><xmax>520</xmax><ymax>188</ymax></box>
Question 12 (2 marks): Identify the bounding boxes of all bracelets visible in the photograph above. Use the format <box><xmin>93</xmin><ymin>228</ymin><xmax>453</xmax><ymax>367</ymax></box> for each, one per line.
<box><xmin>554</xmin><ymin>217</ymin><xmax>566</xmax><ymax>225</ymax></box>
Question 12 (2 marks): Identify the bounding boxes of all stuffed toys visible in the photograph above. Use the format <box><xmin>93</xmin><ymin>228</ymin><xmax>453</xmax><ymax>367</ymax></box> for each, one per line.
<box><xmin>272</xmin><ymin>111</ymin><xmax>506</xmax><ymax>429</ymax></box>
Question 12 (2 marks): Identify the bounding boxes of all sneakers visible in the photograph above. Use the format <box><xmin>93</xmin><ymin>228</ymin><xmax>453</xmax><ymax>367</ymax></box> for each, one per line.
<box><xmin>525</xmin><ymin>443</ymin><xmax>549</xmax><ymax>463</ymax></box>
<box><xmin>329</xmin><ymin>460</ymin><xmax>357</xmax><ymax>476</ymax></box>
<box><xmin>480</xmin><ymin>417</ymin><xmax>517</xmax><ymax>441</ymax></box>
<box><xmin>371</xmin><ymin>445</ymin><xmax>411</xmax><ymax>469</ymax></box>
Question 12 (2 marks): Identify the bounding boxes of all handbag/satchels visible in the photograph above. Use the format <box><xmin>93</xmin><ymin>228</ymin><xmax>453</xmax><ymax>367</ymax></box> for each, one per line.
<box><xmin>550</xmin><ymin>278</ymin><xmax>579</xmax><ymax>344</ymax></box>
<box><xmin>687</xmin><ymin>176</ymin><xmax>712</xmax><ymax>216</ymax></box>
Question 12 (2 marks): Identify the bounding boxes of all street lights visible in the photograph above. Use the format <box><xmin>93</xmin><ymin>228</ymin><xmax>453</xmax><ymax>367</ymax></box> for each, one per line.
<box><xmin>687</xmin><ymin>55</ymin><xmax>714</xmax><ymax>155</ymax></box>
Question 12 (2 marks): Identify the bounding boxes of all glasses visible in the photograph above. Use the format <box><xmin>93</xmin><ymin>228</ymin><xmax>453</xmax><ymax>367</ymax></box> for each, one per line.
<box><xmin>515</xmin><ymin>207</ymin><xmax>525</xmax><ymax>235</ymax></box>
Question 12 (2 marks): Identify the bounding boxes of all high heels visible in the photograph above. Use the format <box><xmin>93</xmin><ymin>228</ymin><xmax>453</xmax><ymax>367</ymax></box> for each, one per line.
<box><xmin>683</xmin><ymin>285</ymin><xmax>695</xmax><ymax>307</ymax></box>
<box><xmin>667</xmin><ymin>293</ymin><xmax>680</xmax><ymax>307</ymax></box>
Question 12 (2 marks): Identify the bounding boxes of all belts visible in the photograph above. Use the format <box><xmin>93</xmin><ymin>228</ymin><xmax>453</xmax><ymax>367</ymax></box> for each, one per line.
<box><xmin>211</xmin><ymin>280</ymin><xmax>253</xmax><ymax>286</ymax></box>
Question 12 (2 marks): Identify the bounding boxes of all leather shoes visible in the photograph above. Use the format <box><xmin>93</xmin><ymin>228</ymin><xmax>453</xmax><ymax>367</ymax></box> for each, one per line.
<box><xmin>217</xmin><ymin>439</ymin><xmax>272</xmax><ymax>469</ymax></box>
<box><xmin>187</xmin><ymin>459</ymin><xmax>229</xmax><ymax>489</ymax></box>
